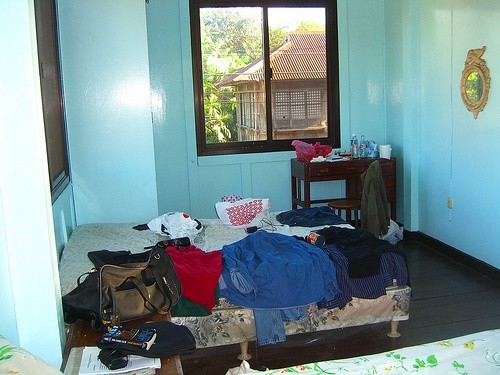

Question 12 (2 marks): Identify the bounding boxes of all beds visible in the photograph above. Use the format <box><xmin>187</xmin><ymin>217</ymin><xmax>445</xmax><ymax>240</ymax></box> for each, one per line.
<box><xmin>58</xmin><ymin>209</ymin><xmax>411</xmax><ymax>361</ymax></box>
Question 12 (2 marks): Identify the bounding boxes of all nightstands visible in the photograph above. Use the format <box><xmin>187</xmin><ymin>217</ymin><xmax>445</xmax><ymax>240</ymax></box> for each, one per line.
<box><xmin>60</xmin><ymin>320</ymin><xmax>184</xmax><ymax>375</ymax></box>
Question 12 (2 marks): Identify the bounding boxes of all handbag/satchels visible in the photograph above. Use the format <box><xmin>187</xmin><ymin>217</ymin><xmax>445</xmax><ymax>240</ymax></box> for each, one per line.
<box><xmin>94</xmin><ymin>261</ymin><xmax>173</xmax><ymax>325</ymax></box>
<box><xmin>87</xmin><ymin>246</ymin><xmax>182</xmax><ymax>309</ymax></box>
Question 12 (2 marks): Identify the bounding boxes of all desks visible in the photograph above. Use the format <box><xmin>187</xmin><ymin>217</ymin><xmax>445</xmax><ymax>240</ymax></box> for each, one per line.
<box><xmin>291</xmin><ymin>157</ymin><xmax>396</xmax><ymax>222</ymax></box>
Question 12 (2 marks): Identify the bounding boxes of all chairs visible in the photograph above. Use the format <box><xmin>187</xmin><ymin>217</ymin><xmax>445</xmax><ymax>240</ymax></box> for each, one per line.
<box><xmin>327</xmin><ymin>160</ymin><xmax>381</xmax><ymax>228</ymax></box>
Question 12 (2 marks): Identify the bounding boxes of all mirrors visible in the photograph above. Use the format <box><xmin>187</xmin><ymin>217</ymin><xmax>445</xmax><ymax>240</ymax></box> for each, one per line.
<box><xmin>460</xmin><ymin>46</ymin><xmax>491</xmax><ymax>119</ymax></box>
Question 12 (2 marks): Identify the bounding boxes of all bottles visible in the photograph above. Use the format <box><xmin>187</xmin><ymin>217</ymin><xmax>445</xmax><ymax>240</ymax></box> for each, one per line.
<box><xmin>360</xmin><ymin>136</ymin><xmax>367</xmax><ymax>157</ymax></box>
<box><xmin>350</xmin><ymin>134</ymin><xmax>357</xmax><ymax>158</ymax></box>
